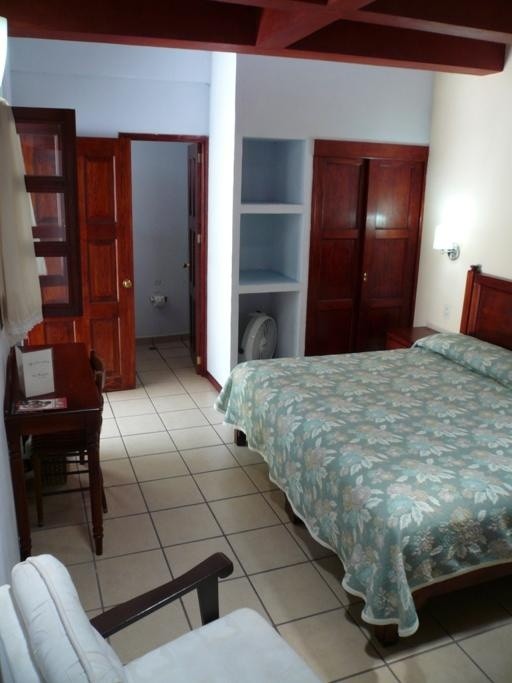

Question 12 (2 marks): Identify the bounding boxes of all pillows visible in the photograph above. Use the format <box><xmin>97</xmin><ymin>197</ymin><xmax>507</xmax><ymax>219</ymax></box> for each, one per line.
<box><xmin>418</xmin><ymin>329</ymin><xmax>512</xmax><ymax>387</ymax></box>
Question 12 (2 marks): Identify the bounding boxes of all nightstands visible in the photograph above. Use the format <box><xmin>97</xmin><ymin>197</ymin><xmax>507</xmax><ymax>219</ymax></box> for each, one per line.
<box><xmin>384</xmin><ymin>324</ymin><xmax>442</xmax><ymax>349</ymax></box>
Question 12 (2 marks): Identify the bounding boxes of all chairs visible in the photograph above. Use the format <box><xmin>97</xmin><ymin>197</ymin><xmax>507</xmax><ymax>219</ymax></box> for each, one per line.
<box><xmin>32</xmin><ymin>350</ymin><xmax>107</xmax><ymax>525</ymax></box>
<box><xmin>1</xmin><ymin>548</ymin><xmax>325</xmax><ymax>681</ymax></box>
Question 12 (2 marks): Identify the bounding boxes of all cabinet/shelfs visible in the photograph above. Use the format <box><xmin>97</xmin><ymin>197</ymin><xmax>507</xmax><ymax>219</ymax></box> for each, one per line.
<box><xmin>23</xmin><ymin>130</ymin><xmax>122</xmax><ymax>390</ymax></box>
<box><xmin>304</xmin><ymin>138</ymin><xmax>430</xmax><ymax>352</ymax></box>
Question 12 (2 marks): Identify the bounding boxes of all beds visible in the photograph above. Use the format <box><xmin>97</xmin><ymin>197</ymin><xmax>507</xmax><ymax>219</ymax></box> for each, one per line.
<box><xmin>232</xmin><ymin>268</ymin><xmax>512</xmax><ymax>643</ymax></box>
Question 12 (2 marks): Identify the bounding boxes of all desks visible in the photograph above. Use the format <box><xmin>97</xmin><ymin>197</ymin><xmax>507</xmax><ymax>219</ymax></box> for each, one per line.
<box><xmin>5</xmin><ymin>341</ymin><xmax>126</xmax><ymax>561</ymax></box>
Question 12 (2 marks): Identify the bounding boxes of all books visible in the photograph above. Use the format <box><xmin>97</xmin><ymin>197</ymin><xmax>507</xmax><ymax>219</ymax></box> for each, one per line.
<box><xmin>12</xmin><ymin>399</ymin><xmax>70</xmax><ymax>414</ymax></box>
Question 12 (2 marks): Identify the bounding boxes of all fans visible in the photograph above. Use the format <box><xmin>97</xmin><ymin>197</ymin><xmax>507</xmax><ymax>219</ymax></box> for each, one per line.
<box><xmin>238</xmin><ymin>308</ymin><xmax>279</xmax><ymax>359</ymax></box>
